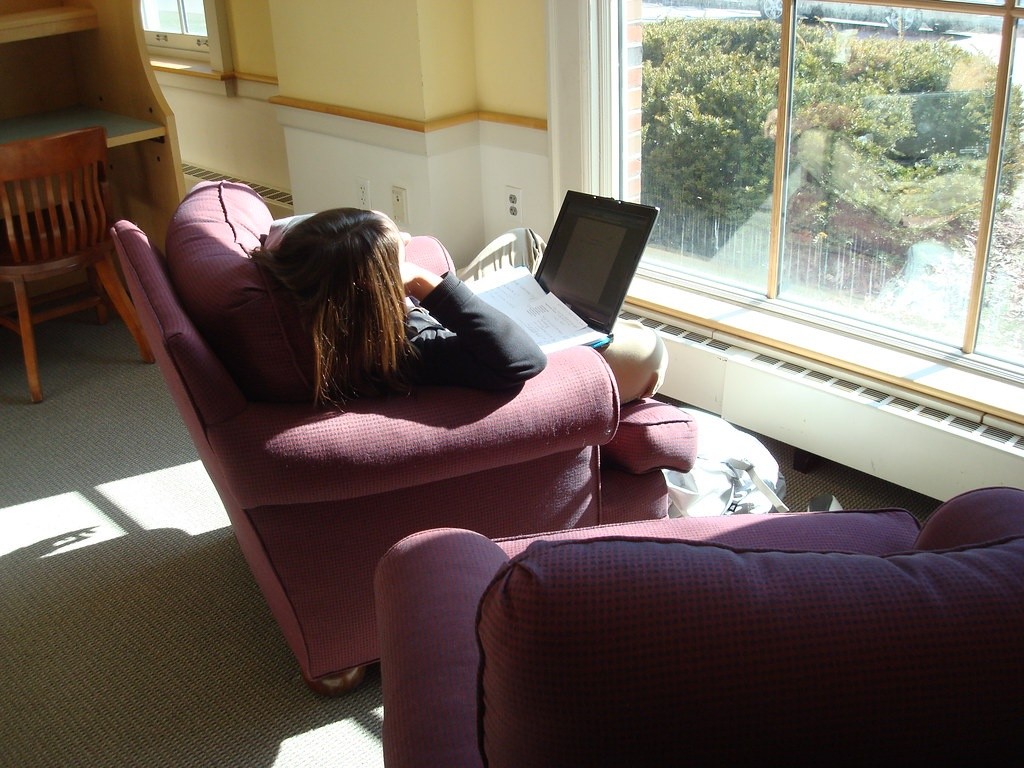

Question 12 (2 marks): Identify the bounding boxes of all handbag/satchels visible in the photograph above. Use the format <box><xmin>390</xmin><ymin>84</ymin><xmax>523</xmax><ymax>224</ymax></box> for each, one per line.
<box><xmin>661</xmin><ymin>407</ymin><xmax>789</xmax><ymax>519</ymax></box>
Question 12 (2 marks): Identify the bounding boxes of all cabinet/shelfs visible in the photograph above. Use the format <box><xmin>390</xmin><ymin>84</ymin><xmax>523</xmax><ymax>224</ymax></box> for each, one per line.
<box><xmin>0</xmin><ymin>0</ymin><xmax>186</xmax><ymax>312</ymax></box>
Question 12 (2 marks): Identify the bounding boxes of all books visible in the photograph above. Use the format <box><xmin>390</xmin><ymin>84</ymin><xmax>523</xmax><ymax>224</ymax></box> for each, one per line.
<box><xmin>464</xmin><ymin>265</ymin><xmax>609</xmax><ymax>355</ymax></box>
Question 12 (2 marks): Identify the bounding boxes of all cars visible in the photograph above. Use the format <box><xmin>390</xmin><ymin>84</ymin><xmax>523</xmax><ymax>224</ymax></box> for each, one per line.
<box><xmin>758</xmin><ymin>0</ymin><xmax>952</xmax><ymax>34</ymax></box>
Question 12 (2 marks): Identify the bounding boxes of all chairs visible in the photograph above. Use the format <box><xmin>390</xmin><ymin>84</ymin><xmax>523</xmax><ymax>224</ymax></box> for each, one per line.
<box><xmin>0</xmin><ymin>126</ymin><xmax>156</xmax><ymax>403</ymax></box>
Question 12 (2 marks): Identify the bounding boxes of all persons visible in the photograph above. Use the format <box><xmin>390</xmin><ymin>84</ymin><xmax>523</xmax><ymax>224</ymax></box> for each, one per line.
<box><xmin>250</xmin><ymin>208</ymin><xmax>668</xmax><ymax>407</ymax></box>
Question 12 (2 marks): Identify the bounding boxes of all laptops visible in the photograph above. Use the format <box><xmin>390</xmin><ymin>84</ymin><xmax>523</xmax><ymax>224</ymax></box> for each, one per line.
<box><xmin>534</xmin><ymin>191</ymin><xmax>661</xmax><ymax>348</ymax></box>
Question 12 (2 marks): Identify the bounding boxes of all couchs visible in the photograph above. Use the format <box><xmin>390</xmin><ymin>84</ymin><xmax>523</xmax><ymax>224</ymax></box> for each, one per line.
<box><xmin>377</xmin><ymin>486</ymin><xmax>1024</xmax><ymax>768</ymax></box>
<box><xmin>110</xmin><ymin>180</ymin><xmax>697</xmax><ymax>698</ymax></box>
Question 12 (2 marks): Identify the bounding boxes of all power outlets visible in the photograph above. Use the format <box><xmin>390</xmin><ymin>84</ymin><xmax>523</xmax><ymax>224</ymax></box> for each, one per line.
<box><xmin>357</xmin><ymin>178</ymin><xmax>370</xmax><ymax>209</ymax></box>
<box><xmin>504</xmin><ymin>185</ymin><xmax>522</xmax><ymax>225</ymax></box>
